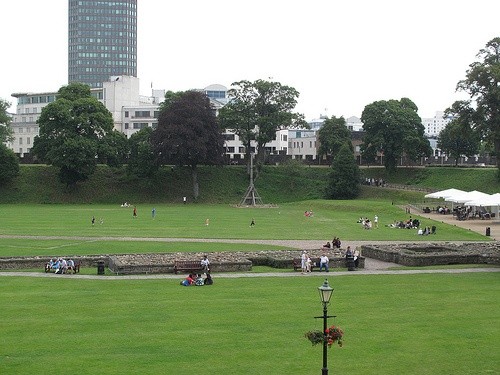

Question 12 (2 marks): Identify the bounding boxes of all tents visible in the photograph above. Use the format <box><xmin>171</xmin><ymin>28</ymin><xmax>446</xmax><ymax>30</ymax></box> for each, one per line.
<box><xmin>424</xmin><ymin>188</ymin><xmax>500</xmax><ymax>220</ymax></box>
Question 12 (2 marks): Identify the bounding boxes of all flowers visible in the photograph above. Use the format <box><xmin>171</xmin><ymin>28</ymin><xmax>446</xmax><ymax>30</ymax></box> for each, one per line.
<box><xmin>325</xmin><ymin>317</ymin><xmax>343</xmax><ymax>348</ymax></box>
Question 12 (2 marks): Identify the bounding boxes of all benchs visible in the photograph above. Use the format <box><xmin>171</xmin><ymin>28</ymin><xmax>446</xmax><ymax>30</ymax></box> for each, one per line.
<box><xmin>45</xmin><ymin>259</ymin><xmax>80</xmax><ymax>274</ymax></box>
<box><xmin>174</xmin><ymin>260</ymin><xmax>211</xmax><ymax>275</ymax></box>
<box><xmin>294</xmin><ymin>257</ymin><xmax>321</xmax><ymax>272</ymax></box>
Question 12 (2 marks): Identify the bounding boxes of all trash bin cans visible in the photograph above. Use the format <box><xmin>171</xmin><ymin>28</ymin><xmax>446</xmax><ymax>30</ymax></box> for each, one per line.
<box><xmin>486</xmin><ymin>227</ymin><xmax>490</xmax><ymax>235</ymax></box>
<box><xmin>357</xmin><ymin>256</ymin><xmax>365</xmax><ymax>267</ymax></box>
<box><xmin>97</xmin><ymin>260</ymin><xmax>104</xmax><ymax>275</ymax></box>
<box><xmin>348</xmin><ymin>258</ymin><xmax>354</xmax><ymax>270</ymax></box>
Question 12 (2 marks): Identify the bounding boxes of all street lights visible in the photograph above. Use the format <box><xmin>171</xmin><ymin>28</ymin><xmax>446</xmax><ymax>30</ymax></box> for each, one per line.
<box><xmin>314</xmin><ymin>278</ymin><xmax>336</xmax><ymax>375</ymax></box>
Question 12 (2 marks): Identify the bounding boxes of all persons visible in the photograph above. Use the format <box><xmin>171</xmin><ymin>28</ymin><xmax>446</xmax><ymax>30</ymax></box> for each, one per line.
<box><xmin>46</xmin><ymin>257</ymin><xmax>75</xmax><ymax>275</ymax></box>
<box><xmin>318</xmin><ymin>253</ymin><xmax>331</xmax><ymax>272</ymax></box>
<box><xmin>183</xmin><ymin>195</ymin><xmax>187</xmax><ymax>204</ymax></box>
<box><xmin>358</xmin><ymin>215</ymin><xmax>379</xmax><ymax>230</ymax></box>
<box><xmin>299</xmin><ymin>250</ymin><xmax>313</xmax><ymax>274</ymax></box>
<box><xmin>360</xmin><ymin>176</ymin><xmax>386</xmax><ymax>188</ymax></box>
<box><xmin>133</xmin><ymin>205</ymin><xmax>138</xmax><ymax>218</ymax></box>
<box><xmin>323</xmin><ymin>236</ymin><xmax>341</xmax><ymax>250</ymax></box>
<box><xmin>249</xmin><ymin>217</ymin><xmax>255</xmax><ymax>228</ymax></box>
<box><xmin>304</xmin><ymin>210</ymin><xmax>314</xmax><ymax>218</ymax></box>
<box><xmin>405</xmin><ymin>208</ymin><xmax>411</xmax><ymax>214</ymax></box>
<box><xmin>90</xmin><ymin>216</ymin><xmax>96</xmax><ymax>228</ymax></box>
<box><xmin>464</xmin><ymin>156</ymin><xmax>468</xmax><ymax>164</ymax></box>
<box><xmin>180</xmin><ymin>254</ymin><xmax>214</xmax><ymax>286</ymax></box>
<box><xmin>475</xmin><ymin>155</ymin><xmax>479</xmax><ymax>163</ymax></box>
<box><xmin>152</xmin><ymin>208</ymin><xmax>156</xmax><ymax>220</ymax></box>
<box><xmin>120</xmin><ymin>200</ymin><xmax>131</xmax><ymax>208</ymax></box>
<box><xmin>346</xmin><ymin>245</ymin><xmax>360</xmax><ymax>271</ymax></box>
<box><xmin>385</xmin><ymin>216</ymin><xmax>437</xmax><ymax>236</ymax></box>
